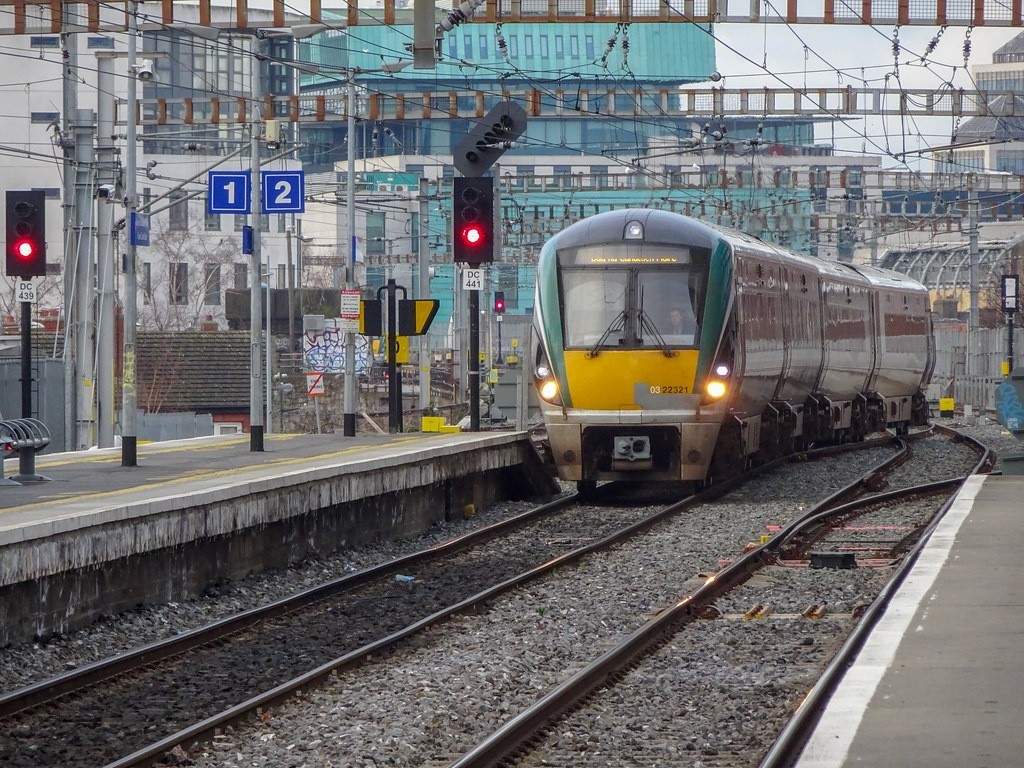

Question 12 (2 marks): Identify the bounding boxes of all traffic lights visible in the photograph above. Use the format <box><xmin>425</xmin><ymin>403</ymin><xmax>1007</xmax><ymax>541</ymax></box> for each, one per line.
<box><xmin>6</xmin><ymin>190</ymin><xmax>46</xmax><ymax>277</ymax></box>
<box><xmin>454</xmin><ymin>177</ymin><xmax>493</xmax><ymax>264</ymax></box>
<box><xmin>495</xmin><ymin>291</ymin><xmax>505</xmax><ymax>312</ymax></box>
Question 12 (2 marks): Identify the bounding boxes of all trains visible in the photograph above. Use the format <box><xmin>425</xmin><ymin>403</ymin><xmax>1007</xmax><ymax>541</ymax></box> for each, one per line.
<box><xmin>529</xmin><ymin>208</ymin><xmax>939</xmax><ymax>498</ymax></box>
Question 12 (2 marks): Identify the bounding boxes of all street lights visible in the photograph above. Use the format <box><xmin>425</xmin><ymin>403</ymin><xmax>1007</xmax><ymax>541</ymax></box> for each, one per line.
<box><xmin>163</xmin><ymin>21</ymin><xmax>346</xmax><ymax>451</ymax></box>
<box><xmin>281</xmin><ymin>60</ymin><xmax>413</xmax><ymax>437</ymax></box>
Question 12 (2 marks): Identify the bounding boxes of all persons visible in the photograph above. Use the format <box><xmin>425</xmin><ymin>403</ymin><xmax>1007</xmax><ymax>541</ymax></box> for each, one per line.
<box><xmin>666</xmin><ymin>309</ymin><xmax>695</xmax><ymax>334</ymax></box>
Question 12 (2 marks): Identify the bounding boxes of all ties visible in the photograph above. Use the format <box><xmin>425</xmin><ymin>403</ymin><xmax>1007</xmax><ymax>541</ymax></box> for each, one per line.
<box><xmin>675</xmin><ymin>328</ymin><xmax>678</xmax><ymax>335</ymax></box>
